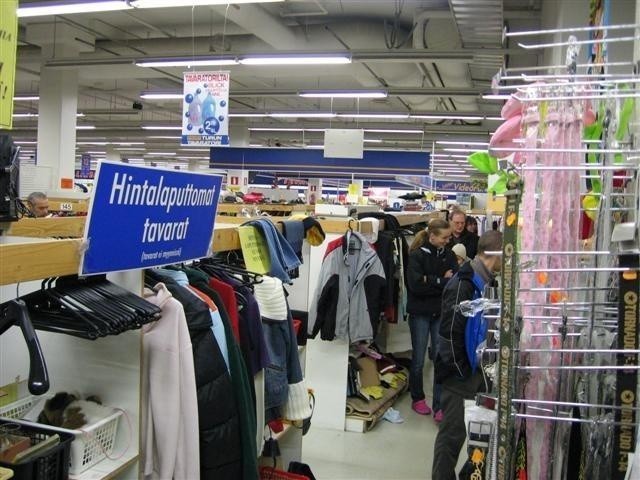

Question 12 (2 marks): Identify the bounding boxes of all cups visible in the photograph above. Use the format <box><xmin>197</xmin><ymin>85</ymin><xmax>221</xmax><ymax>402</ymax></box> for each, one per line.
<box><xmin>0</xmin><ymin>423</ymin><xmax>21</xmax><ymax>449</ymax></box>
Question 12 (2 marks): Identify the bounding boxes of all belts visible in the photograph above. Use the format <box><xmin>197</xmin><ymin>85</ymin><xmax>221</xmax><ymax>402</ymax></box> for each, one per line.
<box><xmin>459</xmin><ymin>38</ymin><xmax>640</xmax><ymax>480</ymax></box>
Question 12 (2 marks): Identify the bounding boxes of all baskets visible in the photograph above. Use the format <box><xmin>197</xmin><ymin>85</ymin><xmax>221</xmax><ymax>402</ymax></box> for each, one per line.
<box><xmin>0</xmin><ymin>393</ymin><xmax>125</xmax><ymax>480</ymax></box>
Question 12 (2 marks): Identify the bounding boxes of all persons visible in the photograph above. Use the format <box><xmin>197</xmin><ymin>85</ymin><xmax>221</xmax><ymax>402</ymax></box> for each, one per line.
<box><xmin>446</xmin><ymin>209</ymin><xmax>480</xmax><ymax>260</ymax></box>
<box><xmin>464</xmin><ymin>216</ymin><xmax>478</xmax><ymax>233</ymax></box>
<box><xmin>405</xmin><ymin>217</ymin><xmax>460</xmax><ymax>423</ymax></box>
<box><xmin>26</xmin><ymin>191</ymin><xmax>49</xmax><ymax>218</ymax></box>
<box><xmin>451</xmin><ymin>243</ymin><xmax>472</xmax><ymax>268</ymax></box>
<box><xmin>430</xmin><ymin>229</ymin><xmax>503</xmax><ymax>480</ymax></box>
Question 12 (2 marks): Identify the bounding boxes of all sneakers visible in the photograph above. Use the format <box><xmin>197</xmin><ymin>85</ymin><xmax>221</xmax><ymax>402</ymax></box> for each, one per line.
<box><xmin>412</xmin><ymin>399</ymin><xmax>442</xmax><ymax>421</ymax></box>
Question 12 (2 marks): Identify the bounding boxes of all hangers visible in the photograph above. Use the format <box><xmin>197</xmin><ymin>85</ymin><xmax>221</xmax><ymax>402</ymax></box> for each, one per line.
<box><xmin>144</xmin><ymin>256</ymin><xmax>267</xmax><ymax>295</ymax></box>
<box><xmin>341</xmin><ymin>219</ymin><xmax>363</xmax><ymax>250</ymax></box>
<box><xmin>1</xmin><ymin>272</ymin><xmax>162</xmax><ymax>395</ymax></box>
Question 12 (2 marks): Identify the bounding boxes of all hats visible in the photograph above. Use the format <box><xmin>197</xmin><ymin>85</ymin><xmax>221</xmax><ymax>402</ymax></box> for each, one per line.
<box><xmin>452</xmin><ymin>244</ymin><xmax>466</xmax><ymax>259</ymax></box>
